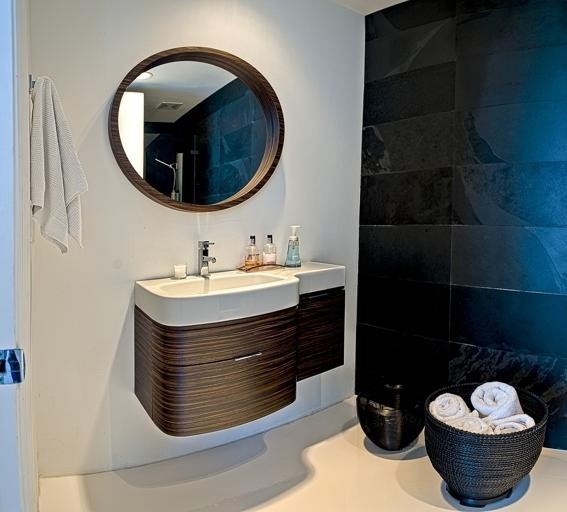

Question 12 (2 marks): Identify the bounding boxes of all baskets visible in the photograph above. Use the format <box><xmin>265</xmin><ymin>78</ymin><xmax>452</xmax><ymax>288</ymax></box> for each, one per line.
<box><xmin>426</xmin><ymin>381</ymin><xmax>548</xmax><ymax>500</ymax></box>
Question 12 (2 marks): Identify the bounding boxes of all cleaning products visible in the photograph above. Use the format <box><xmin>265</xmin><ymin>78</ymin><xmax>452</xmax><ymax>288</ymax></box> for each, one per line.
<box><xmin>245</xmin><ymin>235</ymin><xmax>261</xmax><ymax>266</ymax></box>
<box><xmin>262</xmin><ymin>234</ymin><xmax>277</xmax><ymax>265</ymax></box>
<box><xmin>284</xmin><ymin>225</ymin><xmax>302</xmax><ymax>268</ymax></box>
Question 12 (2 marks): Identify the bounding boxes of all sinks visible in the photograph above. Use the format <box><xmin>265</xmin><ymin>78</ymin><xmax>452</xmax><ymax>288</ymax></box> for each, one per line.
<box><xmin>136</xmin><ymin>272</ymin><xmax>300</xmax><ymax>325</ymax></box>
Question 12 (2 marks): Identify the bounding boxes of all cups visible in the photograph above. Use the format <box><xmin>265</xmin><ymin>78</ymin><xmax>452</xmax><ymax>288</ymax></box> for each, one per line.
<box><xmin>173</xmin><ymin>261</ymin><xmax>187</xmax><ymax>279</ymax></box>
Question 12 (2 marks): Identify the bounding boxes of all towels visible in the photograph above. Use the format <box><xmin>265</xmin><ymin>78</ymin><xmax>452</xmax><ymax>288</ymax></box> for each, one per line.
<box><xmin>428</xmin><ymin>391</ymin><xmax>468</xmax><ymax>427</ymax></box>
<box><xmin>469</xmin><ymin>381</ymin><xmax>524</xmax><ymax>422</ymax></box>
<box><xmin>24</xmin><ymin>71</ymin><xmax>90</xmax><ymax>258</ymax></box>
<box><xmin>488</xmin><ymin>415</ymin><xmax>534</xmax><ymax>437</ymax></box>
<box><xmin>457</xmin><ymin>417</ymin><xmax>490</xmax><ymax>444</ymax></box>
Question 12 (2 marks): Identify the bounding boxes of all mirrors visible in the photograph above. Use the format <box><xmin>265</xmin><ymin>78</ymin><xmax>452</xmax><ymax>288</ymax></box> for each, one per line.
<box><xmin>108</xmin><ymin>47</ymin><xmax>286</xmax><ymax>214</ymax></box>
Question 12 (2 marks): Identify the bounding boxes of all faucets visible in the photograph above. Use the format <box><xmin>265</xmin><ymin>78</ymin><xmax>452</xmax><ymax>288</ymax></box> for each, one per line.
<box><xmin>198</xmin><ymin>240</ymin><xmax>217</xmax><ymax>277</ymax></box>
